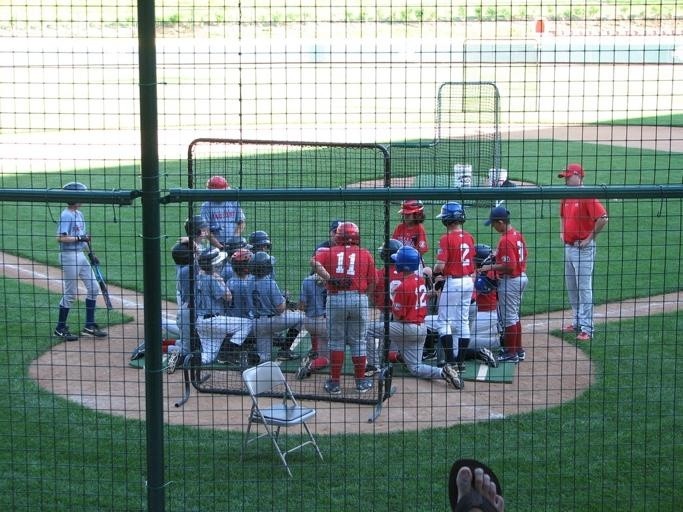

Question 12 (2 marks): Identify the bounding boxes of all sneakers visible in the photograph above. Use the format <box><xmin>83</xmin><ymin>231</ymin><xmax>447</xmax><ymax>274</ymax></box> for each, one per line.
<box><xmin>166</xmin><ymin>348</ymin><xmax>180</xmax><ymax>374</ymax></box>
<box><xmin>131</xmin><ymin>341</ymin><xmax>145</xmax><ymax>360</ymax></box>
<box><xmin>217</xmin><ymin>350</ymin><xmax>249</xmax><ymax>369</ymax></box>
<box><xmin>477</xmin><ymin>345</ymin><xmax>525</xmax><ymax>368</ymax></box>
<box><xmin>80</xmin><ymin>327</ymin><xmax>108</xmax><ymax>338</ymax></box>
<box><xmin>324</xmin><ymin>365</ymin><xmax>381</xmax><ymax>395</ymax></box>
<box><xmin>437</xmin><ymin>360</ymin><xmax>466</xmax><ymax>389</ymax></box>
<box><xmin>276</xmin><ymin>349</ymin><xmax>318</xmax><ymax>380</ymax></box>
<box><xmin>575</xmin><ymin>332</ymin><xmax>593</xmax><ymax>339</ymax></box>
<box><xmin>54</xmin><ymin>326</ymin><xmax>78</xmax><ymax>341</ymax></box>
<box><xmin>560</xmin><ymin>325</ymin><xmax>580</xmax><ymax>334</ymax></box>
<box><xmin>422</xmin><ymin>349</ymin><xmax>438</xmax><ymax>361</ymax></box>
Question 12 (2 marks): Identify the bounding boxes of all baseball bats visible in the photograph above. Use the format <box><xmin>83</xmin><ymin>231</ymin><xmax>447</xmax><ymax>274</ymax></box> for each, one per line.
<box><xmin>85</xmin><ymin>233</ymin><xmax>107</xmax><ymax>291</ymax></box>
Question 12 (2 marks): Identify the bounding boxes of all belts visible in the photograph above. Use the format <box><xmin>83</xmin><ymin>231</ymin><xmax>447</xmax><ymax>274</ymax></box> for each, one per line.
<box><xmin>204</xmin><ymin>313</ymin><xmax>219</xmax><ymax>318</ymax></box>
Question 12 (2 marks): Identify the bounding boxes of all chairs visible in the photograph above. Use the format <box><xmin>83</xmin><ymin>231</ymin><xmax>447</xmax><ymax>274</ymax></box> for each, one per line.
<box><xmin>241</xmin><ymin>361</ymin><xmax>325</xmax><ymax>479</ymax></box>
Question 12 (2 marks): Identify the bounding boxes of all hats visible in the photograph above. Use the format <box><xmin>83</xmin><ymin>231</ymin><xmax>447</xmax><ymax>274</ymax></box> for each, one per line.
<box><xmin>557</xmin><ymin>163</ymin><xmax>585</xmax><ymax>178</ymax></box>
<box><xmin>484</xmin><ymin>206</ymin><xmax>510</xmax><ymax>226</ymax></box>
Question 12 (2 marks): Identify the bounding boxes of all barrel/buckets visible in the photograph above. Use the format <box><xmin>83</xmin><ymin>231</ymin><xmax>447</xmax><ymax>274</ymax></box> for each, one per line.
<box><xmin>489</xmin><ymin>168</ymin><xmax>507</xmax><ymax>186</ymax></box>
<box><xmin>454</xmin><ymin>164</ymin><xmax>472</xmax><ymax>187</ymax></box>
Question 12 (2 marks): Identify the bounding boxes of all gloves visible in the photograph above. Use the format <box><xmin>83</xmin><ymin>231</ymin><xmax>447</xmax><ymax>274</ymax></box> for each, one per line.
<box><xmin>78</xmin><ymin>234</ymin><xmax>90</xmax><ymax>242</ymax></box>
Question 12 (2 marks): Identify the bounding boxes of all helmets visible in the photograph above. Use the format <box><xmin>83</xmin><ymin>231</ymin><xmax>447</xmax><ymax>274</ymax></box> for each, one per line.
<box><xmin>172</xmin><ymin>230</ymin><xmax>276</xmax><ymax>277</ymax></box>
<box><xmin>329</xmin><ymin>220</ymin><xmax>360</xmax><ymax>245</ymax></box>
<box><xmin>436</xmin><ymin>202</ymin><xmax>466</xmax><ymax>226</ymax></box>
<box><xmin>472</xmin><ymin>244</ymin><xmax>496</xmax><ymax>265</ymax></box>
<box><xmin>184</xmin><ymin>216</ymin><xmax>210</xmax><ymax>238</ymax></box>
<box><xmin>377</xmin><ymin>239</ymin><xmax>420</xmax><ymax>273</ymax></box>
<box><xmin>397</xmin><ymin>200</ymin><xmax>425</xmax><ymax>219</ymax></box>
<box><xmin>205</xmin><ymin>175</ymin><xmax>230</xmax><ymax>190</ymax></box>
<box><xmin>62</xmin><ymin>181</ymin><xmax>88</xmax><ymax>206</ymax></box>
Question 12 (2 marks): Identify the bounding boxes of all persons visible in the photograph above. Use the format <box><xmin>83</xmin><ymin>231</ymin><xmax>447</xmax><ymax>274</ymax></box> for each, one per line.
<box><xmin>172</xmin><ymin>176</ymin><xmax>308</xmax><ymax>364</ymax></box>
<box><xmin>557</xmin><ymin>164</ymin><xmax>608</xmax><ymax>341</ymax></box>
<box><xmin>53</xmin><ymin>181</ymin><xmax>108</xmax><ymax>342</ymax></box>
<box><xmin>295</xmin><ymin>200</ymin><xmax>503</xmax><ymax>394</ymax></box>
<box><xmin>479</xmin><ymin>206</ymin><xmax>529</xmax><ymax>363</ymax></box>
